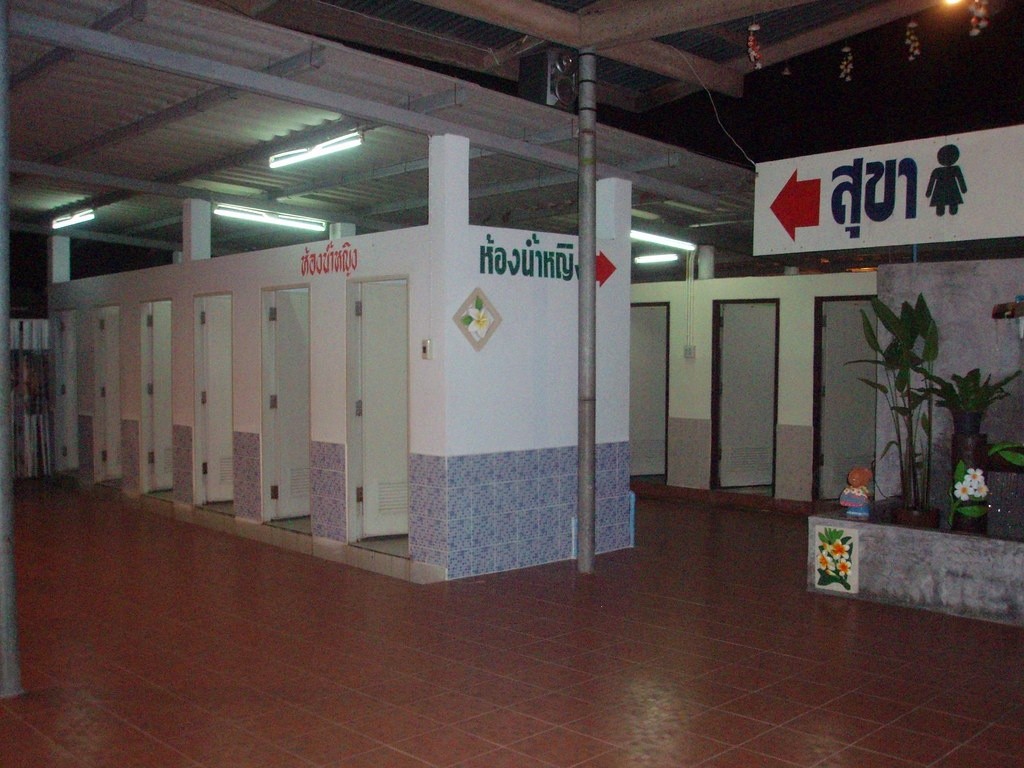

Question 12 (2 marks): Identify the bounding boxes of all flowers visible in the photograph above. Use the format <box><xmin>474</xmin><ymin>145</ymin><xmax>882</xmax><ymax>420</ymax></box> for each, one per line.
<box><xmin>948</xmin><ymin>460</ymin><xmax>990</xmax><ymax>530</ymax></box>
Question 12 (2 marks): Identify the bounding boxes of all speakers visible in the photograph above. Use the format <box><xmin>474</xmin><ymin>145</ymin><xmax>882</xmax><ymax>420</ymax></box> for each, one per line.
<box><xmin>517</xmin><ymin>47</ymin><xmax>579</xmax><ymax>117</ymax></box>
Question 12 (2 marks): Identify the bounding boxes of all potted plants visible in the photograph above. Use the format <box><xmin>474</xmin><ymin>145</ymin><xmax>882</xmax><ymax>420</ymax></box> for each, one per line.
<box><xmin>844</xmin><ymin>292</ymin><xmax>941</xmax><ymax>531</ymax></box>
<box><xmin>918</xmin><ymin>367</ymin><xmax>1023</xmax><ymax>435</ymax></box>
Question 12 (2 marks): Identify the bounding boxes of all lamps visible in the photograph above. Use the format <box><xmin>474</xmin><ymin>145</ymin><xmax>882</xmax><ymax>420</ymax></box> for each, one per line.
<box><xmin>52</xmin><ymin>206</ymin><xmax>97</xmax><ymax>232</ymax></box>
<box><xmin>629</xmin><ymin>225</ymin><xmax>698</xmax><ymax>264</ymax></box>
<box><xmin>269</xmin><ymin>129</ymin><xmax>365</xmax><ymax>168</ymax></box>
<box><xmin>208</xmin><ymin>200</ymin><xmax>327</xmax><ymax>234</ymax></box>
